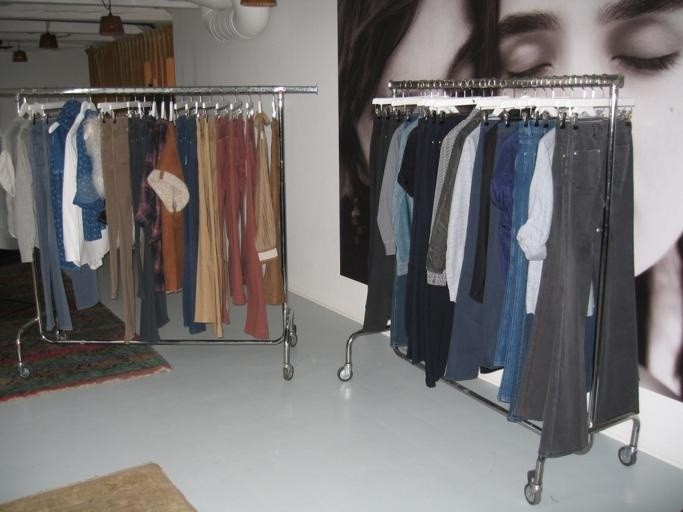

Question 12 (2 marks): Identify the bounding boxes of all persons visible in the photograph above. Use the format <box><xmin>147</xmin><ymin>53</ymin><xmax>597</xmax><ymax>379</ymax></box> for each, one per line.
<box><xmin>486</xmin><ymin>1</ymin><xmax>683</xmax><ymax>402</ymax></box>
<box><xmin>337</xmin><ymin>0</ymin><xmax>492</xmax><ymax>278</ymax></box>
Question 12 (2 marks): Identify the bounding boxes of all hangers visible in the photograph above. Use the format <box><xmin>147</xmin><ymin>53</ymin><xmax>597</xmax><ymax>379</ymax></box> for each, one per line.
<box><xmin>370</xmin><ymin>75</ymin><xmax>635</xmax><ymax>131</ymax></box>
<box><xmin>18</xmin><ymin>87</ymin><xmax>284</xmax><ymax>140</ymax></box>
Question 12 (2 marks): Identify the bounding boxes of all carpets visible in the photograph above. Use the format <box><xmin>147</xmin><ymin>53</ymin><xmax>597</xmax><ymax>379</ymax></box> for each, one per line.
<box><xmin>0</xmin><ymin>245</ymin><xmax>171</xmax><ymax>404</ymax></box>
<box><xmin>1</xmin><ymin>464</ymin><xmax>197</xmax><ymax>512</ymax></box>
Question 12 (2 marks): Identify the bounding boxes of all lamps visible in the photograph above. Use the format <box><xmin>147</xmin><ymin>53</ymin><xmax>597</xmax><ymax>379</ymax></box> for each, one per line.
<box><xmin>39</xmin><ymin>21</ymin><xmax>58</xmax><ymax>49</ymax></box>
<box><xmin>241</xmin><ymin>0</ymin><xmax>277</xmax><ymax>7</ymax></box>
<box><xmin>99</xmin><ymin>0</ymin><xmax>125</xmax><ymax>35</ymax></box>
<box><xmin>12</xmin><ymin>39</ymin><xmax>27</xmax><ymax>62</ymax></box>
